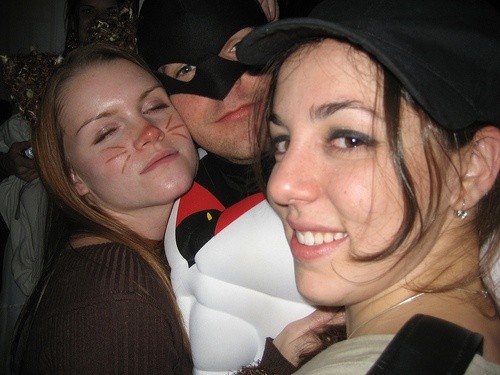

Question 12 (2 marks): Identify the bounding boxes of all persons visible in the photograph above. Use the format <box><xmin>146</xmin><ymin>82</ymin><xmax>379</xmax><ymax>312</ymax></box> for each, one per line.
<box><xmin>0</xmin><ymin>0</ymin><xmax>499</xmax><ymax>375</ymax></box>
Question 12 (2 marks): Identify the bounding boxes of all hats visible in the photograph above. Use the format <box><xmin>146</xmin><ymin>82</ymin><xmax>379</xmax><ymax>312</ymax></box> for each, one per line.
<box><xmin>235</xmin><ymin>0</ymin><xmax>500</xmax><ymax>131</ymax></box>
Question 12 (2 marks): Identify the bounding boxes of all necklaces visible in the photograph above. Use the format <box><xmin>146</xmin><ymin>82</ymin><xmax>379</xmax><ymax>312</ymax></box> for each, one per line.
<box><xmin>346</xmin><ymin>287</ymin><xmax>490</xmax><ymax>340</ymax></box>
